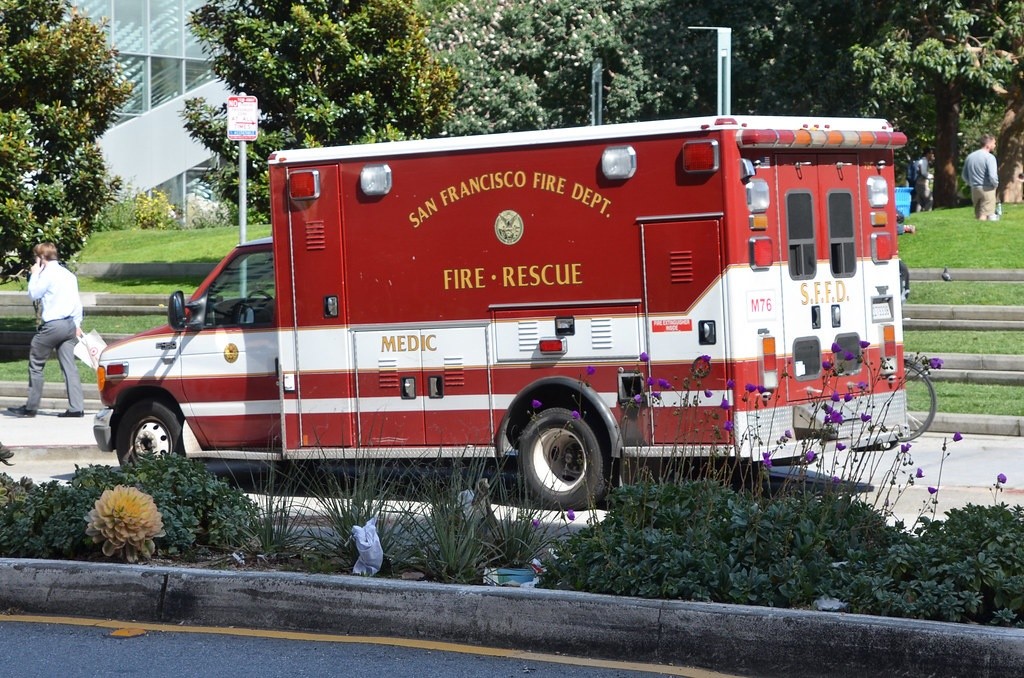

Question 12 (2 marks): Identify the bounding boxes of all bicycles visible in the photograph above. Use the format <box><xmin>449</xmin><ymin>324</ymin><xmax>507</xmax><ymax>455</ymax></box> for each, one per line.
<box><xmin>901</xmin><ymin>356</ymin><xmax>937</xmax><ymax>444</ymax></box>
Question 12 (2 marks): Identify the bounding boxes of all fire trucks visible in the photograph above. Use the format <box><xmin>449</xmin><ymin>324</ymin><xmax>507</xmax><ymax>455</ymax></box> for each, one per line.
<box><xmin>91</xmin><ymin>111</ymin><xmax>913</xmax><ymax>515</ymax></box>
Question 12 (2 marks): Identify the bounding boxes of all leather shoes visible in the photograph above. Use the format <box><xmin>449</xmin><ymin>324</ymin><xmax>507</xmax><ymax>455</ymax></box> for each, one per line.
<box><xmin>8</xmin><ymin>405</ymin><xmax>37</xmax><ymax>418</ymax></box>
<box><xmin>58</xmin><ymin>410</ymin><xmax>84</xmax><ymax>417</ymax></box>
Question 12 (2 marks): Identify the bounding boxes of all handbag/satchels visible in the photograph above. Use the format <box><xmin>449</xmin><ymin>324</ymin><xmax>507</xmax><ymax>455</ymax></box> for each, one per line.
<box><xmin>73</xmin><ymin>329</ymin><xmax>107</xmax><ymax>371</ymax></box>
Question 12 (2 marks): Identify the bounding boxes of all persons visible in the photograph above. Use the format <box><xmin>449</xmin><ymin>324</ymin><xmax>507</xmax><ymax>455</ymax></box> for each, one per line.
<box><xmin>897</xmin><ymin>223</ymin><xmax>917</xmax><ymax>235</ymax></box>
<box><xmin>913</xmin><ymin>146</ymin><xmax>934</xmax><ymax>212</ymax></box>
<box><xmin>8</xmin><ymin>242</ymin><xmax>86</xmax><ymax>418</ymax></box>
<box><xmin>961</xmin><ymin>134</ymin><xmax>999</xmax><ymax>220</ymax></box>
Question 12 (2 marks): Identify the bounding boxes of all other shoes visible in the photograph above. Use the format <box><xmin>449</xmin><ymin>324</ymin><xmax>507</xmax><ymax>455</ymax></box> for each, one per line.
<box><xmin>904</xmin><ymin>224</ymin><xmax>915</xmax><ymax>233</ymax></box>
